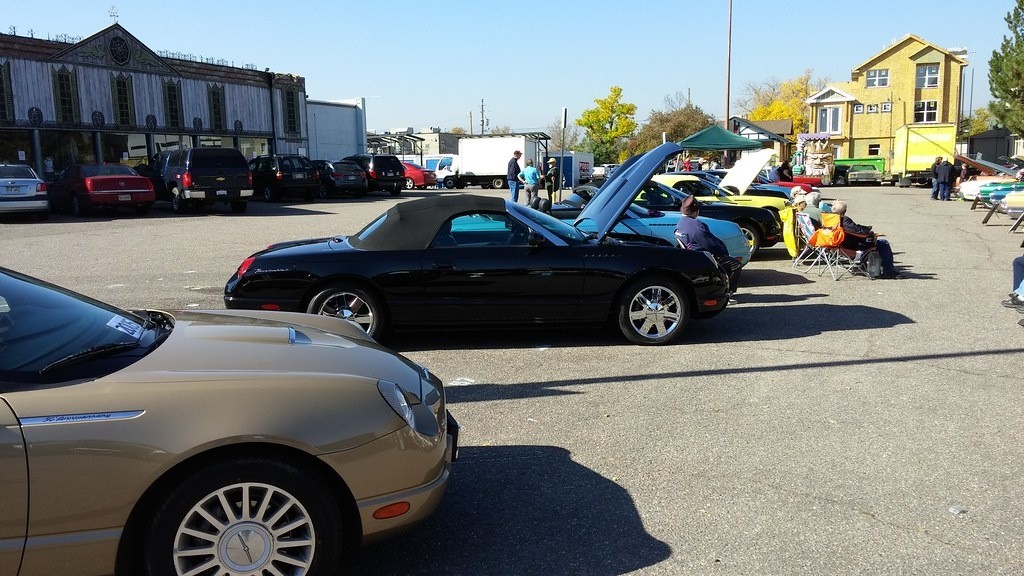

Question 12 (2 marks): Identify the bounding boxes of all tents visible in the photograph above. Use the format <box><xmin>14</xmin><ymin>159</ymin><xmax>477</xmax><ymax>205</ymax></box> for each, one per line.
<box><xmin>675</xmin><ymin>125</ymin><xmax>762</xmax><ymax>170</ymax></box>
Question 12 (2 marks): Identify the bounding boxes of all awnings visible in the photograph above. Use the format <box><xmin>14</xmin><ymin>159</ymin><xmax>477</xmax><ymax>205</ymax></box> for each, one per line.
<box><xmin>797</xmin><ymin>133</ymin><xmax>830</xmax><ymax>141</ymax></box>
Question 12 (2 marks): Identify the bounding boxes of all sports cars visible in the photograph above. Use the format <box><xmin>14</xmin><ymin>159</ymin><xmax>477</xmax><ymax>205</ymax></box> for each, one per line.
<box><xmin>451</xmin><ymin>185</ymin><xmax>751</xmax><ymax>268</ymax></box>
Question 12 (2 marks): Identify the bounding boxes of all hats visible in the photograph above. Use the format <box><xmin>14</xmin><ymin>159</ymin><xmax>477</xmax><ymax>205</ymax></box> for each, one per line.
<box><xmin>514</xmin><ymin>151</ymin><xmax>522</xmax><ymax>154</ymax></box>
<box><xmin>547</xmin><ymin>158</ymin><xmax>556</xmax><ymax>164</ymax></box>
<box><xmin>794</xmin><ymin>195</ymin><xmax>806</xmax><ymax>204</ymax></box>
<box><xmin>791</xmin><ymin>185</ymin><xmax>807</xmax><ymax>194</ymax></box>
<box><xmin>680</xmin><ymin>195</ymin><xmax>702</xmax><ymax>214</ymax></box>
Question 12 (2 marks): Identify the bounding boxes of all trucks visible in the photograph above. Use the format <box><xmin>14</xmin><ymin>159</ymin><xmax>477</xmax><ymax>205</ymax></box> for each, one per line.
<box><xmin>889</xmin><ymin>121</ymin><xmax>957</xmax><ymax>186</ymax></box>
<box><xmin>435</xmin><ymin>137</ymin><xmax>537</xmax><ymax>189</ymax></box>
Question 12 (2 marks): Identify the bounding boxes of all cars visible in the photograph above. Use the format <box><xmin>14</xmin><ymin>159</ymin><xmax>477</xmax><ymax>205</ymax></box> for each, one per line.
<box><xmin>523</xmin><ymin>142</ymin><xmax>822</xmax><ymax>269</ymax></box>
<box><xmin>845</xmin><ymin>163</ymin><xmax>882</xmax><ymax>185</ymax></box>
<box><xmin>0</xmin><ymin>164</ymin><xmax>51</xmax><ymax>219</ymax></box>
<box><xmin>1</xmin><ymin>265</ymin><xmax>459</xmax><ymax>576</ymax></box>
<box><xmin>63</xmin><ymin>162</ymin><xmax>156</xmax><ymax>217</ymax></box>
<box><xmin>956</xmin><ymin>155</ymin><xmax>1024</xmax><ymax>217</ymax></box>
<box><xmin>400</xmin><ymin>161</ymin><xmax>437</xmax><ymax>190</ymax></box>
<box><xmin>223</xmin><ymin>142</ymin><xmax>731</xmax><ymax>346</ymax></box>
<box><xmin>312</xmin><ymin>158</ymin><xmax>369</xmax><ymax>198</ymax></box>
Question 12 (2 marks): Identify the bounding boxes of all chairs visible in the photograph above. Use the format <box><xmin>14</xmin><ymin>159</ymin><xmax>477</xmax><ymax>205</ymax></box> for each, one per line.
<box><xmin>530</xmin><ymin>196</ymin><xmax>552</xmax><ymax>217</ymax></box>
<box><xmin>437</xmin><ymin>220</ymin><xmax>456</xmax><ymax>248</ymax></box>
<box><xmin>791</xmin><ymin>211</ymin><xmax>883</xmax><ymax>281</ymax></box>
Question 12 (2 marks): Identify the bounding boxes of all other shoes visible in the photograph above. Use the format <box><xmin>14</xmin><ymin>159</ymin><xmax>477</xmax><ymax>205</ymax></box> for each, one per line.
<box><xmin>1002</xmin><ymin>297</ymin><xmax>1024</xmax><ymax>308</ymax></box>
<box><xmin>931</xmin><ymin>197</ymin><xmax>939</xmax><ymax>200</ymax></box>
<box><xmin>883</xmin><ymin>270</ymin><xmax>905</xmax><ymax>279</ymax></box>
<box><xmin>728</xmin><ymin>299</ymin><xmax>737</xmax><ymax>305</ymax></box>
<box><xmin>1016</xmin><ymin>306</ymin><xmax>1024</xmax><ymax>313</ymax></box>
<box><xmin>1008</xmin><ymin>292</ymin><xmax>1019</xmax><ymax>298</ymax></box>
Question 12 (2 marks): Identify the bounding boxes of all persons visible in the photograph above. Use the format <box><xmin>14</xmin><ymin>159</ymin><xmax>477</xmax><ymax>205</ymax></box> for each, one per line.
<box><xmin>930</xmin><ymin>156</ymin><xmax>958</xmax><ymax>201</ymax></box>
<box><xmin>507</xmin><ymin>150</ymin><xmax>523</xmax><ymax>202</ymax></box>
<box><xmin>787</xmin><ymin>186</ymin><xmax>905</xmax><ymax>279</ymax></box>
<box><xmin>711</xmin><ymin>158</ymin><xmax>720</xmax><ymax>170</ymax></box>
<box><xmin>1001</xmin><ymin>240</ymin><xmax>1024</xmax><ymax>326</ymax></box>
<box><xmin>517</xmin><ymin>158</ymin><xmax>540</xmax><ymax>205</ymax></box>
<box><xmin>777</xmin><ymin>160</ymin><xmax>793</xmax><ymax>182</ymax></box>
<box><xmin>676</xmin><ymin>194</ymin><xmax>742</xmax><ymax>305</ymax></box>
<box><xmin>684</xmin><ymin>153</ymin><xmax>692</xmax><ymax>172</ymax></box>
<box><xmin>544</xmin><ymin>158</ymin><xmax>560</xmax><ymax>205</ymax></box>
<box><xmin>959</xmin><ymin>163</ymin><xmax>969</xmax><ymax>200</ymax></box>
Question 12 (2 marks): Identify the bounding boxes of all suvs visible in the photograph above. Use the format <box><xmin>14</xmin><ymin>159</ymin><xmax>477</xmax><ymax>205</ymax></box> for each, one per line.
<box><xmin>141</xmin><ymin>147</ymin><xmax>254</xmax><ymax>214</ymax></box>
<box><xmin>250</xmin><ymin>153</ymin><xmax>321</xmax><ymax>202</ymax></box>
<box><xmin>340</xmin><ymin>154</ymin><xmax>406</xmax><ymax>191</ymax></box>
